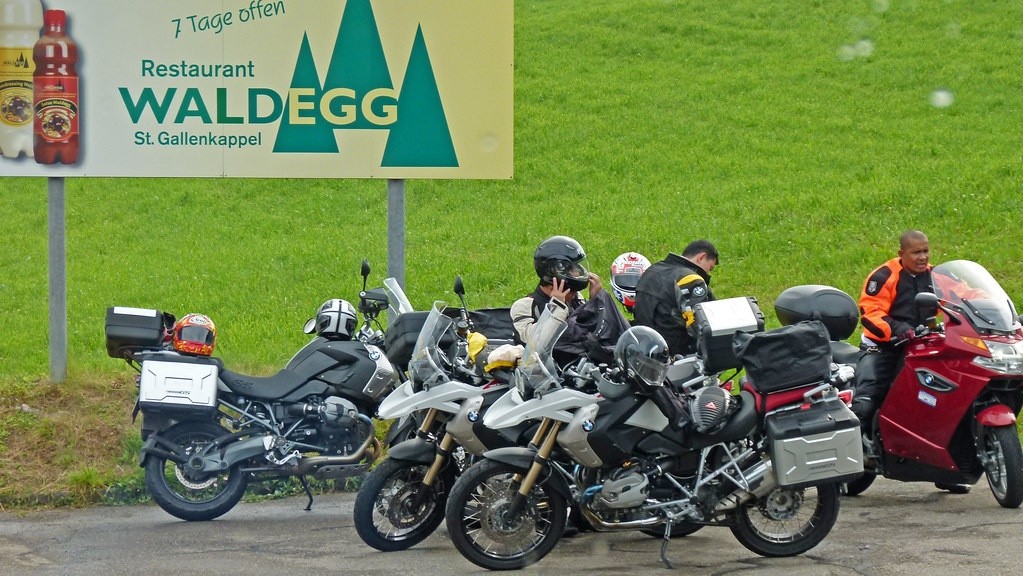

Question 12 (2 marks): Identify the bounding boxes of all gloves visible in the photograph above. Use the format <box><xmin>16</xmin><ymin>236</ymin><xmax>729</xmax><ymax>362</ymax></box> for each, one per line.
<box><xmin>892</xmin><ymin>322</ymin><xmax>916</xmax><ymax>341</ymax></box>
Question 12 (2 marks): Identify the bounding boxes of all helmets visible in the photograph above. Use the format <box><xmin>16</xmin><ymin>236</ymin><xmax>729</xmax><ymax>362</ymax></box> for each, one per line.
<box><xmin>316</xmin><ymin>299</ymin><xmax>358</xmax><ymax>340</ymax></box>
<box><xmin>173</xmin><ymin>314</ymin><xmax>217</xmax><ymax>355</ymax></box>
<box><xmin>611</xmin><ymin>252</ymin><xmax>651</xmax><ymax>307</ymax></box>
<box><xmin>534</xmin><ymin>236</ymin><xmax>589</xmax><ymax>291</ymax></box>
<box><xmin>615</xmin><ymin>325</ymin><xmax>668</xmax><ymax>395</ymax></box>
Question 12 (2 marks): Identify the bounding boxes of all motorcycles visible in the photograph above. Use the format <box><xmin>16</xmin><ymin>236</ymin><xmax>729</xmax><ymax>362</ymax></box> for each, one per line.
<box><xmin>105</xmin><ymin>259</ymin><xmax>1022</xmax><ymax>570</ymax></box>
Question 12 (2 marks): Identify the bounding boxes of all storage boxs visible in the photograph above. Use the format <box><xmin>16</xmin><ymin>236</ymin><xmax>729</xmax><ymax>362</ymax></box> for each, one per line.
<box><xmin>104</xmin><ymin>306</ymin><xmax>218</xmax><ymax>420</ymax></box>
<box><xmin>385</xmin><ymin>312</ymin><xmax>455</xmax><ymax>365</ymax></box>
<box><xmin>774</xmin><ymin>285</ymin><xmax>860</xmax><ymax>343</ymax></box>
<box><xmin>765</xmin><ymin>400</ymin><xmax>865</xmax><ymax>490</ymax></box>
<box><xmin>693</xmin><ymin>295</ymin><xmax>764</xmax><ymax>373</ymax></box>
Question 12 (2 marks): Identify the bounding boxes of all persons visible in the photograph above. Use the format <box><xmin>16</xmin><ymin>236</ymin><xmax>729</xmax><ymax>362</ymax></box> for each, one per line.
<box><xmin>509</xmin><ymin>237</ymin><xmax>603</xmax><ymax>537</ymax></box>
<box><xmin>634</xmin><ymin>240</ymin><xmax>719</xmax><ymax>357</ymax></box>
<box><xmin>851</xmin><ymin>231</ymin><xmax>991</xmax><ymax>494</ymax></box>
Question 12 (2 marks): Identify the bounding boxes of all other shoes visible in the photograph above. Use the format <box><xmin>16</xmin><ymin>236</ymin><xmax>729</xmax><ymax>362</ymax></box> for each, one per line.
<box><xmin>935</xmin><ymin>480</ymin><xmax>971</xmax><ymax>493</ymax></box>
<box><xmin>535</xmin><ymin>510</ymin><xmax>578</xmax><ymax>536</ymax></box>
<box><xmin>568</xmin><ymin>508</ymin><xmax>619</xmax><ymax>531</ymax></box>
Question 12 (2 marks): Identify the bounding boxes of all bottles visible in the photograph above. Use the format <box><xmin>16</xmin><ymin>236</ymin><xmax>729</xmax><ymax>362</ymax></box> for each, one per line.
<box><xmin>33</xmin><ymin>10</ymin><xmax>79</xmax><ymax>164</ymax></box>
<box><xmin>0</xmin><ymin>1</ymin><xmax>44</xmax><ymax>158</ymax></box>
